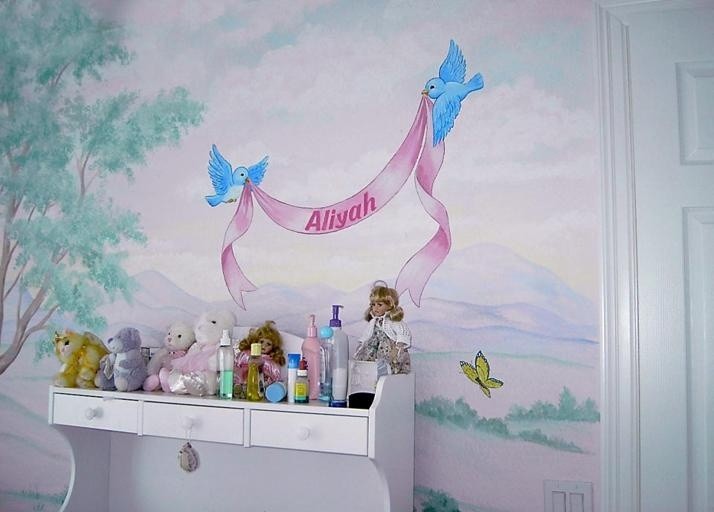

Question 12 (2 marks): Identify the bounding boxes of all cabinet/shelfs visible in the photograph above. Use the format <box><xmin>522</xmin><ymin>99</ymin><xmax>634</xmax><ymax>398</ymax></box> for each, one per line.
<box><xmin>46</xmin><ymin>328</ymin><xmax>416</xmax><ymax>509</ymax></box>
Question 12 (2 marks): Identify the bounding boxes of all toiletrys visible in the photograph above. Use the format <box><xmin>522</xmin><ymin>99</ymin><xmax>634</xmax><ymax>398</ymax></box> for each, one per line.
<box><xmin>217</xmin><ymin>328</ymin><xmax>233</xmax><ymax>396</ymax></box>
<box><xmin>266</xmin><ymin>304</ymin><xmax>350</xmax><ymax>409</ymax></box>
<box><xmin>245</xmin><ymin>338</ymin><xmax>266</xmax><ymax>400</ymax></box>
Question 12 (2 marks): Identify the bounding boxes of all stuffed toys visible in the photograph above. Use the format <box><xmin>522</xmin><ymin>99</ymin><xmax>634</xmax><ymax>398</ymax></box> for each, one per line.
<box><xmin>146</xmin><ymin>322</ymin><xmax>196</xmax><ymax>394</ymax></box>
<box><xmin>93</xmin><ymin>327</ymin><xmax>147</xmax><ymax>396</ymax></box>
<box><xmin>170</xmin><ymin>307</ymin><xmax>233</xmax><ymax>396</ymax></box>
<box><xmin>52</xmin><ymin>329</ymin><xmax>107</xmax><ymax>390</ymax></box>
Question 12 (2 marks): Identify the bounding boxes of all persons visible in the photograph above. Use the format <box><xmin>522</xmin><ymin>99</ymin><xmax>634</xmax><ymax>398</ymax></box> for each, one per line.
<box><xmin>233</xmin><ymin>321</ymin><xmax>283</xmax><ymax>402</ymax></box>
<box><xmin>352</xmin><ymin>284</ymin><xmax>411</xmax><ymax>407</ymax></box>
